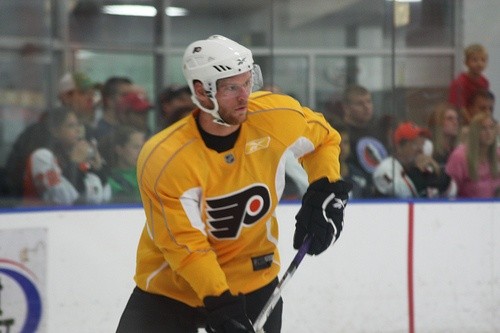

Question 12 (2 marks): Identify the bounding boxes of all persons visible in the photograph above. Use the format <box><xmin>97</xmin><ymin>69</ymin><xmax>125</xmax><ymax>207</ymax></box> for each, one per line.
<box><xmin>0</xmin><ymin>41</ymin><xmax>500</xmax><ymax>206</ymax></box>
<box><xmin>116</xmin><ymin>35</ymin><xmax>352</xmax><ymax>333</ymax></box>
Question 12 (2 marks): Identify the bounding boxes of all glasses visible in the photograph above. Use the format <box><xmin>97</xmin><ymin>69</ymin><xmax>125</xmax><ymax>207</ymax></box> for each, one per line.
<box><xmin>182</xmin><ymin>34</ymin><xmax>255</xmax><ymax>127</ymax></box>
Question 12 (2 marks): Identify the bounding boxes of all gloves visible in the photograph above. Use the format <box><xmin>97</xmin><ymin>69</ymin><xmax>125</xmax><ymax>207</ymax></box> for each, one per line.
<box><xmin>293</xmin><ymin>175</ymin><xmax>349</xmax><ymax>255</ymax></box>
<box><xmin>203</xmin><ymin>289</ymin><xmax>255</xmax><ymax>332</ymax></box>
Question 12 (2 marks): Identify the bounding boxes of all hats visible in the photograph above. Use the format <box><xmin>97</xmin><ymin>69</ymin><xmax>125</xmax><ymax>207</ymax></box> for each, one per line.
<box><xmin>158</xmin><ymin>83</ymin><xmax>193</xmax><ymax>110</ymax></box>
<box><xmin>113</xmin><ymin>91</ymin><xmax>154</xmax><ymax>112</ymax></box>
<box><xmin>393</xmin><ymin>121</ymin><xmax>431</xmax><ymax>144</ymax></box>
<box><xmin>54</xmin><ymin>68</ymin><xmax>104</xmax><ymax>97</ymax></box>
